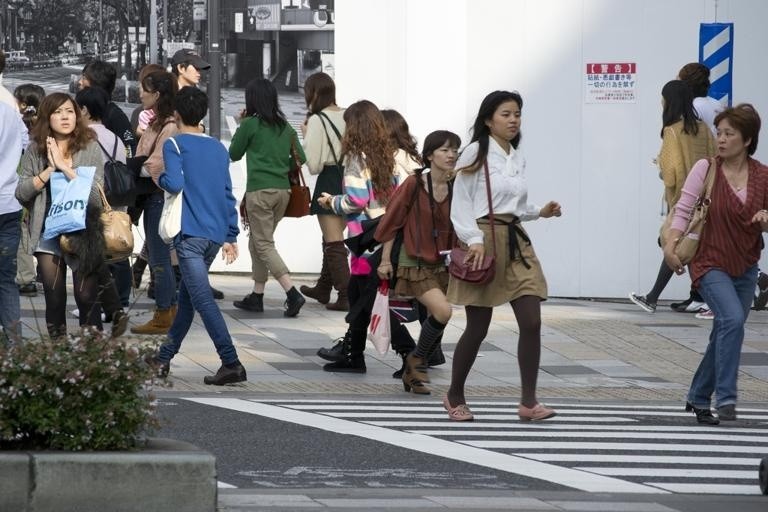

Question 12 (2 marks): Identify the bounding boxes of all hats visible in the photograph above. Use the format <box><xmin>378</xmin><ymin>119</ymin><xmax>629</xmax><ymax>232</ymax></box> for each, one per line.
<box><xmin>171</xmin><ymin>48</ymin><xmax>211</xmax><ymax>71</ymax></box>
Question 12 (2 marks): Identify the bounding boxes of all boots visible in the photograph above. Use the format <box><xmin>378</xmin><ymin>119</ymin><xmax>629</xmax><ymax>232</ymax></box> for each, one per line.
<box><xmin>284</xmin><ymin>286</ymin><xmax>305</xmax><ymax>318</ymax></box>
<box><xmin>300</xmin><ymin>238</ymin><xmax>332</xmax><ymax>305</ymax></box>
<box><xmin>322</xmin><ymin>333</ymin><xmax>367</xmax><ymax>373</ymax></box>
<box><xmin>233</xmin><ymin>292</ymin><xmax>264</xmax><ymax>313</ymax></box>
<box><xmin>130</xmin><ymin>308</ymin><xmax>171</xmax><ymax>334</ymax></box>
<box><xmin>428</xmin><ymin>342</ymin><xmax>446</xmax><ymax>366</ymax></box>
<box><xmin>325</xmin><ymin>241</ymin><xmax>352</xmax><ymax>310</ymax></box>
<box><xmin>316</xmin><ymin>341</ymin><xmax>343</xmax><ymax>361</ymax></box>
<box><xmin>391</xmin><ymin>324</ymin><xmax>417</xmax><ymax>379</ymax></box>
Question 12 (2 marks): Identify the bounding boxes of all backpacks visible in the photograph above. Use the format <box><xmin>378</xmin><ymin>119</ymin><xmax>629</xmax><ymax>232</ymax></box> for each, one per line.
<box><xmin>95</xmin><ymin>135</ymin><xmax>139</xmax><ymax>207</ymax></box>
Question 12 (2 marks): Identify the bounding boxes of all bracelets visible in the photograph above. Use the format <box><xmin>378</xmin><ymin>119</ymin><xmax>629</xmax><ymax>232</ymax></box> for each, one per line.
<box><xmin>378</xmin><ymin>264</ymin><xmax>392</xmax><ymax>267</ymax></box>
<box><xmin>326</xmin><ymin>195</ymin><xmax>334</xmax><ymax>210</ymax></box>
<box><xmin>37</xmin><ymin>174</ymin><xmax>48</xmax><ymax>186</ymax></box>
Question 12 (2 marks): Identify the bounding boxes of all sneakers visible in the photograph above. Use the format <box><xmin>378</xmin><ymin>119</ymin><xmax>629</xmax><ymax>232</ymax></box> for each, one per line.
<box><xmin>111</xmin><ymin>312</ymin><xmax>128</xmax><ymax>339</ymax></box>
<box><xmin>203</xmin><ymin>365</ymin><xmax>246</xmax><ymax>387</ymax></box>
<box><xmin>694</xmin><ymin>311</ymin><xmax>715</xmax><ymax>320</ymax></box>
<box><xmin>629</xmin><ymin>292</ymin><xmax>656</xmax><ymax>313</ymax></box>
<box><xmin>670</xmin><ymin>298</ymin><xmax>709</xmax><ymax>313</ymax></box>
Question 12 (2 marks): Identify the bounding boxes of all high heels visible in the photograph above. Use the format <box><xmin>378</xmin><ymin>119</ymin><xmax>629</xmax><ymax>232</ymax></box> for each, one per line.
<box><xmin>716</xmin><ymin>404</ymin><xmax>736</xmax><ymax>421</ymax></box>
<box><xmin>405</xmin><ymin>353</ymin><xmax>430</xmax><ymax>384</ymax></box>
<box><xmin>684</xmin><ymin>401</ymin><xmax>718</xmax><ymax>426</ymax></box>
<box><xmin>402</xmin><ymin>370</ymin><xmax>431</xmax><ymax>395</ymax></box>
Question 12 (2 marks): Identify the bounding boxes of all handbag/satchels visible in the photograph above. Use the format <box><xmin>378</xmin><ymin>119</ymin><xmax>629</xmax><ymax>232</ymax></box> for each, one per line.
<box><xmin>59</xmin><ymin>179</ymin><xmax>134</xmax><ymax>258</ymax></box>
<box><xmin>658</xmin><ymin>158</ymin><xmax>713</xmax><ymax>265</ymax></box>
<box><xmin>156</xmin><ymin>137</ymin><xmax>184</xmax><ymax>244</ymax></box>
<box><xmin>367</xmin><ymin>278</ymin><xmax>393</xmax><ymax>357</ymax></box>
<box><xmin>42</xmin><ymin>166</ymin><xmax>97</xmax><ymax>242</ymax></box>
<box><xmin>448</xmin><ymin>161</ymin><xmax>496</xmax><ymax>284</ymax></box>
<box><xmin>285</xmin><ymin>137</ymin><xmax>311</xmax><ymax>217</ymax></box>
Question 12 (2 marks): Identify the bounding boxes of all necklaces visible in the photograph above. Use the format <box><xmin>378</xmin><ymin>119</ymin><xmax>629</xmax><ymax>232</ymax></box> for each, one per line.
<box><xmin>732</xmin><ymin>181</ymin><xmax>745</xmax><ymax>191</ymax></box>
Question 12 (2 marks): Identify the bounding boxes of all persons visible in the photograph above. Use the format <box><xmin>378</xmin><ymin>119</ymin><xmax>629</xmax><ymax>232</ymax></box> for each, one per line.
<box><xmin>75</xmin><ymin>86</ymin><xmax>136</xmax><ymax>339</ymax></box>
<box><xmin>299</xmin><ymin>73</ymin><xmax>353</xmax><ymax>312</ymax></box>
<box><xmin>130</xmin><ymin>63</ymin><xmax>184</xmax><ymax>289</ymax></box>
<box><xmin>371</xmin><ymin>131</ymin><xmax>462</xmax><ymax>396</ymax></box>
<box><xmin>148</xmin><ymin>49</ymin><xmax>226</xmax><ymax>302</ymax></box>
<box><xmin>130</xmin><ymin>71</ymin><xmax>182</xmax><ymax>335</ymax></box>
<box><xmin>0</xmin><ymin>49</ymin><xmax>22</xmax><ymax>114</ymax></box>
<box><xmin>0</xmin><ymin>100</ymin><xmax>32</xmax><ymax>345</ymax></box>
<box><xmin>15</xmin><ymin>94</ymin><xmax>106</xmax><ymax>341</ymax></box>
<box><xmin>663</xmin><ymin>102</ymin><xmax>768</xmax><ymax>426</ymax></box>
<box><xmin>314</xmin><ymin>99</ymin><xmax>417</xmax><ymax>380</ymax></box>
<box><xmin>317</xmin><ymin>110</ymin><xmax>448</xmax><ymax>367</ymax></box>
<box><xmin>13</xmin><ymin>84</ymin><xmax>50</xmax><ymax>298</ymax></box>
<box><xmin>443</xmin><ymin>89</ymin><xmax>563</xmax><ymax>424</ymax></box>
<box><xmin>228</xmin><ymin>77</ymin><xmax>307</xmax><ymax>318</ymax></box>
<box><xmin>73</xmin><ymin>59</ymin><xmax>137</xmax><ymax>322</ymax></box>
<box><xmin>144</xmin><ymin>86</ymin><xmax>246</xmax><ymax>386</ymax></box>
<box><xmin>671</xmin><ymin>62</ymin><xmax>768</xmax><ymax>315</ymax></box>
<box><xmin>628</xmin><ymin>77</ymin><xmax>718</xmax><ymax>314</ymax></box>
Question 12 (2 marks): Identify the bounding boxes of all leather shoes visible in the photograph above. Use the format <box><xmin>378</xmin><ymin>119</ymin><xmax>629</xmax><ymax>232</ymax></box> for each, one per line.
<box><xmin>211</xmin><ymin>287</ymin><xmax>225</xmax><ymax>300</ymax></box>
<box><xmin>442</xmin><ymin>394</ymin><xmax>474</xmax><ymax>421</ymax></box>
<box><xmin>518</xmin><ymin>402</ymin><xmax>557</xmax><ymax>422</ymax></box>
<box><xmin>19</xmin><ymin>284</ymin><xmax>37</xmax><ymax>297</ymax></box>
